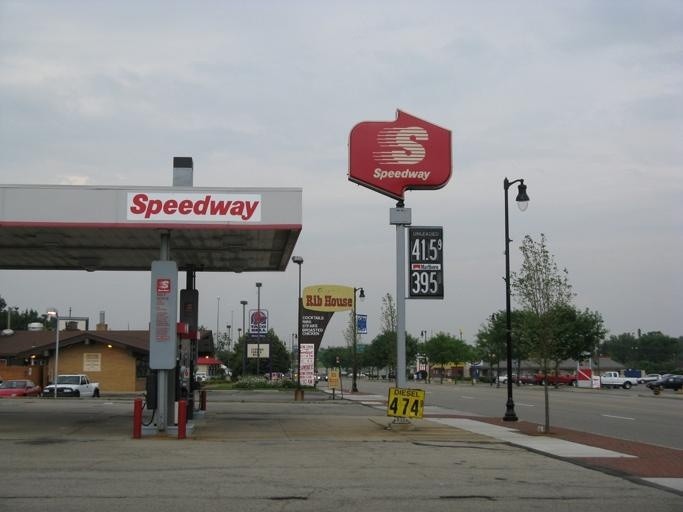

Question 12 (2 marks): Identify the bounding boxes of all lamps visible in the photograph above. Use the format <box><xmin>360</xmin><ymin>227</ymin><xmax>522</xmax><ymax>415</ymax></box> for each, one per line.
<box><xmin>36</xmin><ymin>233</ymin><xmax>104</xmax><ymax>271</ymax></box>
<box><xmin>222</xmin><ymin>234</ymin><xmax>249</xmax><ymax>273</ymax></box>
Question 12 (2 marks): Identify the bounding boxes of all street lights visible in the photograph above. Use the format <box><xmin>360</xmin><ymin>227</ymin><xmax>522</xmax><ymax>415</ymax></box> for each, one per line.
<box><xmin>215</xmin><ymin>294</ymin><xmax>249</xmax><ymax>379</ymax></box>
<box><xmin>254</xmin><ymin>281</ymin><xmax>262</xmax><ymax>377</ymax></box>
<box><xmin>48</xmin><ymin>310</ymin><xmax>59</xmax><ymax>398</ymax></box>
<box><xmin>292</xmin><ymin>255</ymin><xmax>304</xmax><ymax>392</ymax></box>
<box><xmin>502</xmin><ymin>175</ymin><xmax>529</xmax><ymax>423</ymax></box>
<box><xmin>420</xmin><ymin>330</ymin><xmax>426</xmax><ymax>383</ymax></box>
<box><xmin>351</xmin><ymin>287</ymin><xmax>365</xmax><ymax>393</ymax></box>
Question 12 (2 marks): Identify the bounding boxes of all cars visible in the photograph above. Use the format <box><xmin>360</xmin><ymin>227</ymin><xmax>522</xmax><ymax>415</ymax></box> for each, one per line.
<box><xmin>494</xmin><ymin>369</ymin><xmax>682</xmax><ymax>392</ymax></box>
<box><xmin>317</xmin><ymin>370</ymin><xmax>426</xmax><ymax>382</ymax></box>
<box><xmin>0</xmin><ymin>379</ymin><xmax>42</xmax><ymax>398</ymax></box>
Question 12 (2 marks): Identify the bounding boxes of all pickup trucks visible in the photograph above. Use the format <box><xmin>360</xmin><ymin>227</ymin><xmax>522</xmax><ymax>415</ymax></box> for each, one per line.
<box><xmin>43</xmin><ymin>374</ymin><xmax>100</xmax><ymax>398</ymax></box>
